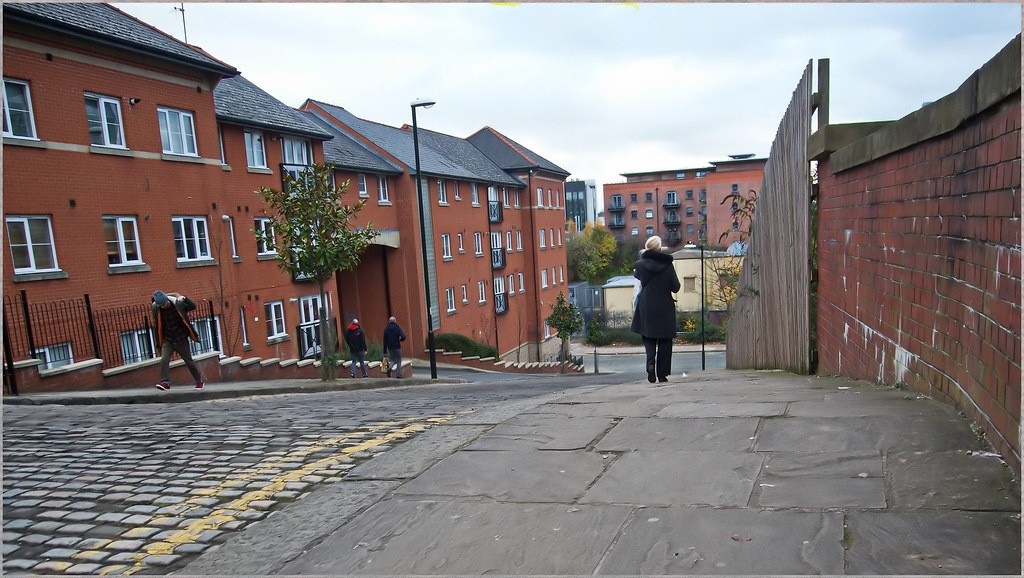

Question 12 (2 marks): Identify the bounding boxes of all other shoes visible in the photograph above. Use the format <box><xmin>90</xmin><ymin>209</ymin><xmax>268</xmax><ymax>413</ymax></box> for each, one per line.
<box><xmin>397</xmin><ymin>376</ymin><xmax>404</xmax><ymax>379</ymax></box>
<box><xmin>388</xmin><ymin>376</ymin><xmax>392</xmax><ymax>378</ymax></box>
<box><xmin>350</xmin><ymin>376</ymin><xmax>355</xmax><ymax>379</ymax></box>
<box><xmin>362</xmin><ymin>376</ymin><xmax>369</xmax><ymax>378</ymax></box>
<box><xmin>657</xmin><ymin>370</ymin><xmax>669</xmax><ymax>382</ymax></box>
<box><xmin>646</xmin><ymin>365</ymin><xmax>657</xmax><ymax>383</ymax></box>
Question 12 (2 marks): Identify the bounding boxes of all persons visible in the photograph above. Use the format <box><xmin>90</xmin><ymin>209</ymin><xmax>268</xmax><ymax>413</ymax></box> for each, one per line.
<box><xmin>383</xmin><ymin>317</ymin><xmax>406</xmax><ymax>378</ymax></box>
<box><xmin>622</xmin><ymin>236</ymin><xmax>680</xmax><ymax>383</ymax></box>
<box><xmin>344</xmin><ymin>319</ymin><xmax>369</xmax><ymax>378</ymax></box>
<box><xmin>152</xmin><ymin>289</ymin><xmax>205</xmax><ymax>391</ymax></box>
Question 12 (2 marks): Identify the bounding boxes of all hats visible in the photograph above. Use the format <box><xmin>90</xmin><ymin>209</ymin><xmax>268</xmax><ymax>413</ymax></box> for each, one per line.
<box><xmin>153</xmin><ymin>290</ymin><xmax>167</xmax><ymax>304</ymax></box>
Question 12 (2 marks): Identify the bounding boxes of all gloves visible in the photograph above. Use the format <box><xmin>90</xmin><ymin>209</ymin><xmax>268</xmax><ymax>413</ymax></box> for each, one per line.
<box><xmin>177</xmin><ymin>295</ymin><xmax>185</xmax><ymax>302</ymax></box>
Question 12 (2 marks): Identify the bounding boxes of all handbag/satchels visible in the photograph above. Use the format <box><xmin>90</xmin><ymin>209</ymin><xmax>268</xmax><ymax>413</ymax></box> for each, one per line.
<box><xmin>380</xmin><ymin>356</ymin><xmax>390</xmax><ymax>374</ymax></box>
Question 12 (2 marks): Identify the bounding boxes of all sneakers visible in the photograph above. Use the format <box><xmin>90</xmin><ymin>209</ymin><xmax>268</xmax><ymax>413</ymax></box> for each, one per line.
<box><xmin>156</xmin><ymin>382</ymin><xmax>170</xmax><ymax>391</ymax></box>
<box><xmin>193</xmin><ymin>377</ymin><xmax>207</xmax><ymax>389</ymax></box>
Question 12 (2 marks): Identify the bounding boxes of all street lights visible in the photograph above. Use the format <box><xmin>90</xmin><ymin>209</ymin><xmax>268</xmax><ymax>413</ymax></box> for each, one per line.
<box><xmin>410</xmin><ymin>100</ymin><xmax>438</xmax><ymax>379</ymax></box>
<box><xmin>685</xmin><ymin>241</ymin><xmax>705</xmax><ymax>370</ymax></box>
